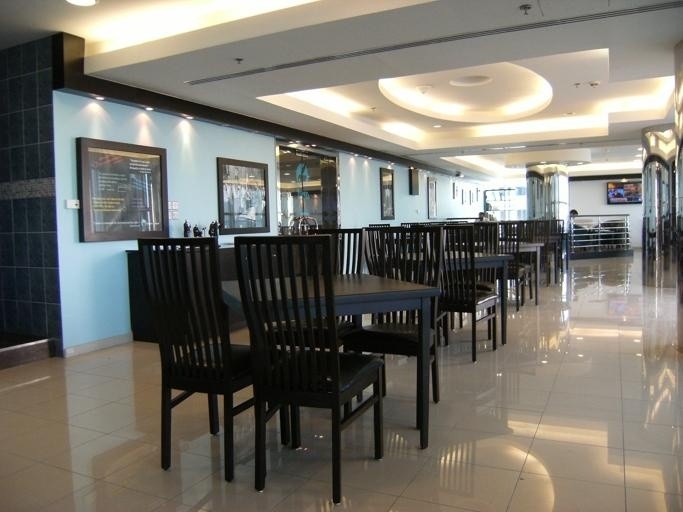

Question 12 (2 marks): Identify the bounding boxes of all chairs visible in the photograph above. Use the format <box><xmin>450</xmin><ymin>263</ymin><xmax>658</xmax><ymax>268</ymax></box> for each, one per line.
<box><xmin>341</xmin><ymin>229</ymin><xmax>444</xmax><ymax>427</ymax></box>
<box><xmin>139</xmin><ymin>237</ymin><xmax>288</xmax><ymax>480</ymax></box>
<box><xmin>235</xmin><ymin>235</ymin><xmax>386</xmax><ymax>503</ymax></box>
<box><xmin>366</xmin><ymin>218</ymin><xmax>568</xmax><ymax>367</ymax></box>
<box><xmin>264</xmin><ymin>229</ymin><xmax>363</xmax><ymax>421</ymax></box>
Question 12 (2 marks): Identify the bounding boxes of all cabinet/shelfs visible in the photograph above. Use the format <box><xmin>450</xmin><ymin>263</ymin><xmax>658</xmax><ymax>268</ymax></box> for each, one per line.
<box><xmin>122</xmin><ymin>242</ymin><xmax>301</xmax><ymax>345</ymax></box>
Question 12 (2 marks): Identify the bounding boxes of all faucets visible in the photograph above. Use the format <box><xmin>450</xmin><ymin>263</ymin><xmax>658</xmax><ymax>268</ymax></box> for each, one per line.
<box><xmin>292</xmin><ymin>215</ymin><xmax>319</xmax><ymax>235</ymax></box>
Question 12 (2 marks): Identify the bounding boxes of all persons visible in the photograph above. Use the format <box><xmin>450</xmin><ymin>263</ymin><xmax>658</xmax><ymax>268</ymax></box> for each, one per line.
<box><xmin>612</xmin><ymin>190</ymin><xmax>620</xmax><ymax>198</ymax></box>
<box><xmin>569</xmin><ymin>210</ymin><xmax>583</xmax><ymax>228</ymax></box>
<box><xmin>474</xmin><ymin>212</ymin><xmax>490</xmax><ymax>222</ymax></box>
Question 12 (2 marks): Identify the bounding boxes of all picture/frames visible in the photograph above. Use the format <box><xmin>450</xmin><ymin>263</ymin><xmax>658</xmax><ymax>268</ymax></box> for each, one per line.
<box><xmin>76</xmin><ymin>136</ymin><xmax>169</xmax><ymax>243</ymax></box>
<box><xmin>427</xmin><ymin>177</ymin><xmax>437</xmax><ymax>219</ymax></box>
<box><xmin>217</xmin><ymin>157</ymin><xmax>270</xmax><ymax>234</ymax></box>
<box><xmin>380</xmin><ymin>167</ymin><xmax>395</xmax><ymax>219</ymax></box>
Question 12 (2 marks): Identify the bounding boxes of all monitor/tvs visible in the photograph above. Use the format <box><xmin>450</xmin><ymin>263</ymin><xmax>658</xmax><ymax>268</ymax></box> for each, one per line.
<box><xmin>607</xmin><ymin>181</ymin><xmax>642</xmax><ymax>204</ymax></box>
